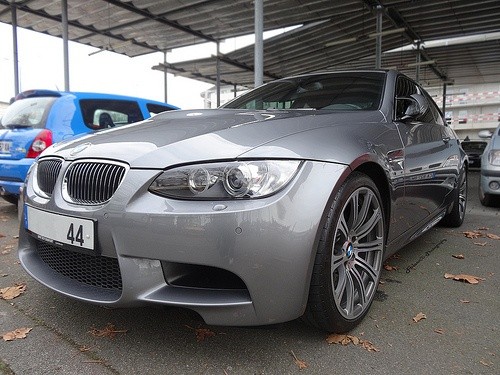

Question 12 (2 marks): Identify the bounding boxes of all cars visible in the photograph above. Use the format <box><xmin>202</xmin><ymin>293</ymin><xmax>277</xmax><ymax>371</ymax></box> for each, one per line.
<box><xmin>479</xmin><ymin>123</ymin><xmax>500</xmax><ymax>207</ymax></box>
<box><xmin>460</xmin><ymin>140</ymin><xmax>488</xmax><ymax>168</ymax></box>
<box><xmin>18</xmin><ymin>68</ymin><xmax>469</xmax><ymax>334</ymax></box>
<box><xmin>1</xmin><ymin>88</ymin><xmax>182</xmax><ymax>205</ymax></box>
